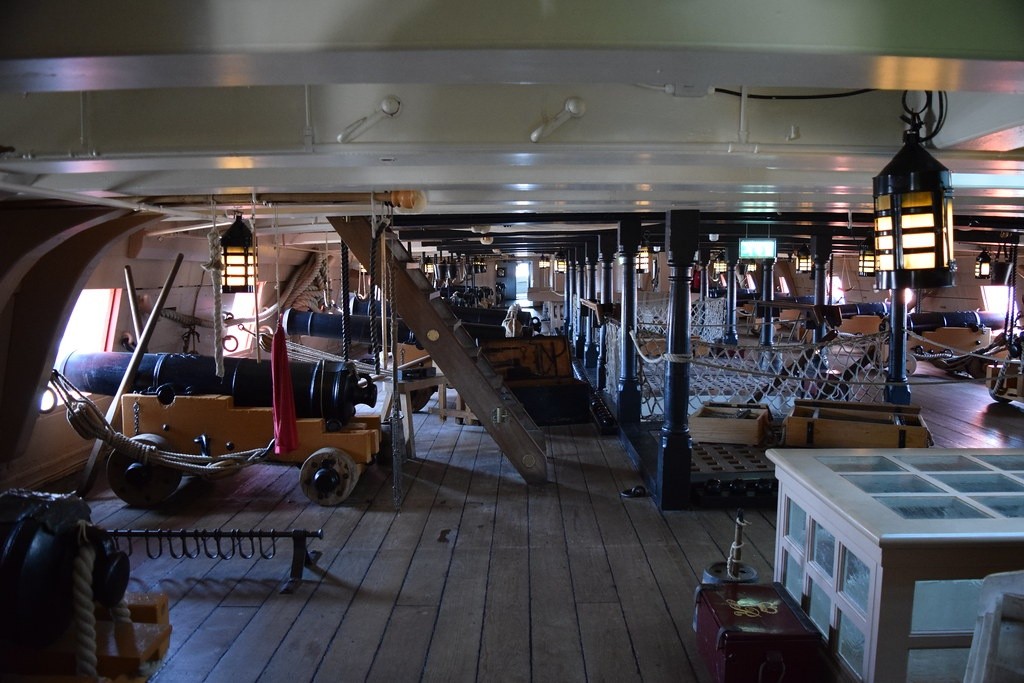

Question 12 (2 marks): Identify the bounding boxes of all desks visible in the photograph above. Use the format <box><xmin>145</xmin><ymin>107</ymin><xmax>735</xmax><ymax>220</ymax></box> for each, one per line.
<box><xmin>766</xmin><ymin>448</ymin><xmax>1024</xmax><ymax>683</ymax></box>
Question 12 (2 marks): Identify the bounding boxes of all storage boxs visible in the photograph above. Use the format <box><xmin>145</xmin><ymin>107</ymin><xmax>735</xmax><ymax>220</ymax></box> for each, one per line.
<box><xmin>782</xmin><ymin>405</ymin><xmax>935</xmax><ymax>450</ymax></box>
<box><xmin>704</xmin><ymin>402</ymin><xmax>774</xmax><ymax>425</ymax></box>
<box><xmin>692</xmin><ymin>582</ymin><xmax>823</xmax><ymax>683</ymax></box>
<box><xmin>688</xmin><ymin>406</ymin><xmax>774</xmax><ymax>447</ymax></box>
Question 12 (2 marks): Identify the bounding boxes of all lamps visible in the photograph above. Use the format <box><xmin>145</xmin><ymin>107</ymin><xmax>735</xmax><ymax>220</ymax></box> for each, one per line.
<box><xmin>974</xmin><ymin>247</ymin><xmax>991</xmax><ymax>279</ymax></box>
<box><xmin>794</xmin><ymin>242</ymin><xmax>813</xmax><ymax>274</ymax></box>
<box><xmin>555</xmin><ymin>247</ymin><xmax>567</xmax><ymax>273</ymax></box>
<box><xmin>858</xmin><ymin>233</ymin><xmax>875</xmax><ymax>278</ymax></box>
<box><xmin>713</xmin><ymin>234</ymin><xmax>728</xmax><ymax>274</ymax></box>
<box><xmin>635</xmin><ymin>232</ymin><xmax>649</xmax><ymax>274</ymax></box>
<box><xmin>872</xmin><ymin>109</ymin><xmax>955</xmax><ymax>291</ymax></box>
